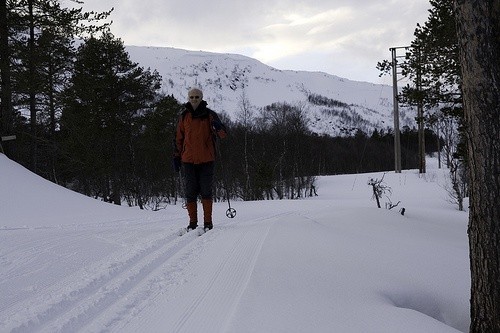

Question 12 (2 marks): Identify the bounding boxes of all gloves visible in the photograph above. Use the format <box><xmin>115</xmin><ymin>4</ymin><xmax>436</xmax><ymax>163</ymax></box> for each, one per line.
<box><xmin>173</xmin><ymin>159</ymin><xmax>182</xmax><ymax>172</ymax></box>
<box><xmin>211</xmin><ymin>120</ymin><xmax>220</xmax><ymax>131</ymax></box>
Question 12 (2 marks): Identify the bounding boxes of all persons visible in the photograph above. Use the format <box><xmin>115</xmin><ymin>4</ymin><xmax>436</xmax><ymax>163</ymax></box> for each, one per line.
<box><xmin>176</xmin><ymin>88</ymin><xmax>225</xmax><ymax>236</ymax></box>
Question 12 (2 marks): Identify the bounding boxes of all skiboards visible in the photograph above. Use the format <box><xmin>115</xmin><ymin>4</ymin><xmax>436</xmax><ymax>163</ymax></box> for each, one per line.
<box><xmin>179</xmin><ymin>229</ymin><xmax>190</xmax><ymax>237</ymax></box>
<box><xmin>197</xmin><ymin>230</ymin><xmax>207</xmax><ymax>236</ymax></box>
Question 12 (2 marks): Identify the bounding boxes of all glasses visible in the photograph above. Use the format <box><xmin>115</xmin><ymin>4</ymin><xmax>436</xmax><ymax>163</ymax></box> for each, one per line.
<box><xmin>190</xmin><ymin>95</ymin><xmax>200</xmax><ymax>99</ymax></box>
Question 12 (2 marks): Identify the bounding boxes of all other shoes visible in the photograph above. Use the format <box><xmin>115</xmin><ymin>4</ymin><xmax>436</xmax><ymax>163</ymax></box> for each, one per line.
<box><xmin>203</xmin><ymin>223</ymin><xmax>213</xmax><ymax>232</ymax></box>
<box><xmin>187</xmin><ymin>222</ymin><xmax>198</xmax><ymax>231</ymax></box>
